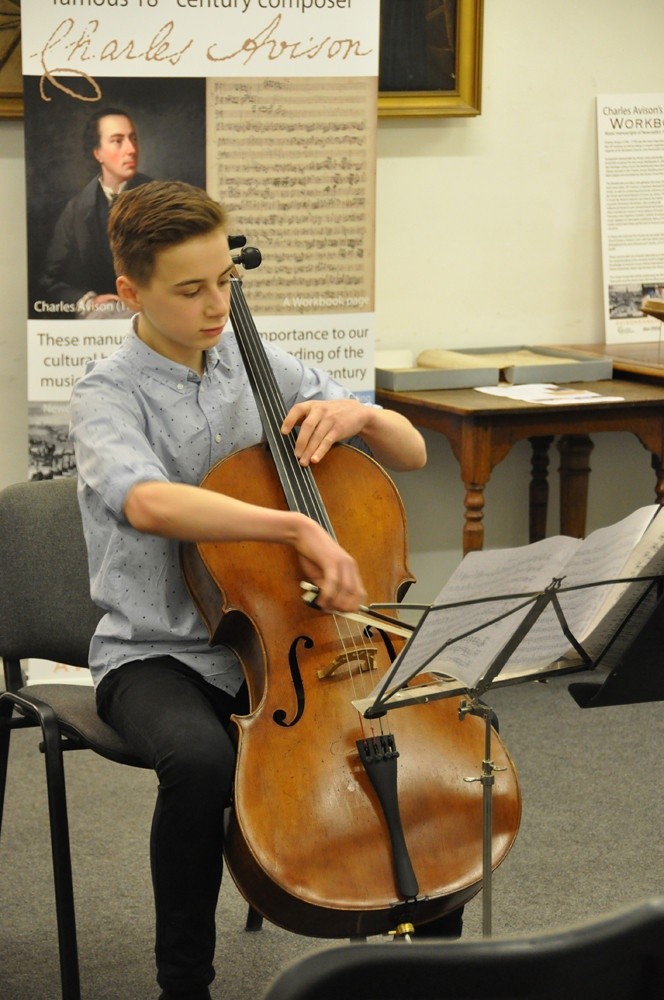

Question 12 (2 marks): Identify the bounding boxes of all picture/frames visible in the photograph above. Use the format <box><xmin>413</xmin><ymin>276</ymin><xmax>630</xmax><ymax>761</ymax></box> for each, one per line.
<box><xmin>378</xmin><ymin>2</ymin><xmax>485</xmax><ymax>119</ymax></box>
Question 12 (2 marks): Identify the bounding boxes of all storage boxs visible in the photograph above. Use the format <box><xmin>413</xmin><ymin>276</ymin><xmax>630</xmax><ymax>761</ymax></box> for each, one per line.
<box><xmin>373</xmin><ymin>361</ymin><xmax>500</xmax><ymax>392</ymax></box>
<box><xmin>444</xmin><ymin>345</ymin><xmax>615</xmax><ymax>385</ymax></box>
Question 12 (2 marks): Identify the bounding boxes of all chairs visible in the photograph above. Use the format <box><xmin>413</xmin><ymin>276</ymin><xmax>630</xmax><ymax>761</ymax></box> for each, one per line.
<box><xmin>0</xmin><ymin>479</ymin><xmax>263</xmax><ymax>1000</ymax></box>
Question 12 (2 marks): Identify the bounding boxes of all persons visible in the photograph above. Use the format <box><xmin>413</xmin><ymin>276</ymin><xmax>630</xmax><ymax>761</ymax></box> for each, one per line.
<box><xmin>66</xmin><ymin>179</ymin><xmax>464</xmax><ymax>999</ymax></box>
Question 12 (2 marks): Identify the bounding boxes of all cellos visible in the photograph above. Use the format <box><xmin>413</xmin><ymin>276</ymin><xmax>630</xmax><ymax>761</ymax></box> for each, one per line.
<box><xmin>179</xmin><ymin>229</ymin><xmax>523</xmax><ymax>946</ymax></box>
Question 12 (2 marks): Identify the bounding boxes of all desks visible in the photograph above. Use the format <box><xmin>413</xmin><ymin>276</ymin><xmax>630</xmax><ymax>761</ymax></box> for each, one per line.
<box><xmin>551</xmin><ymin>343</ymin><xmax>663</xmax><ymax>380</ymax></box>
<box><xmin>376</xmin><ymin>376</ymin><xmax>663</xmax><ymax>569</ymax></box>
